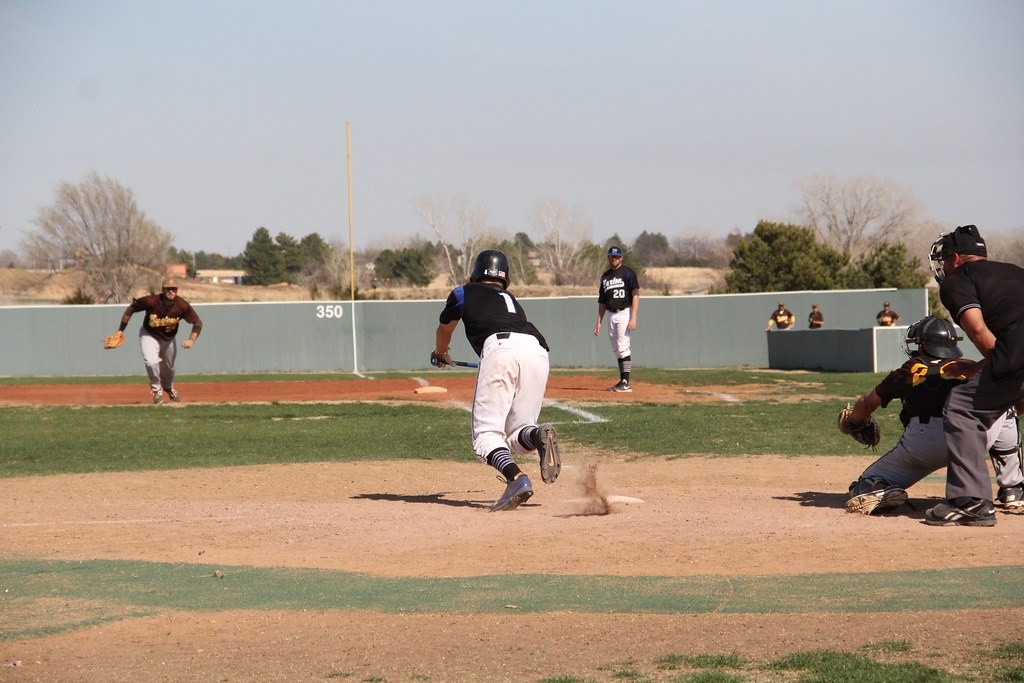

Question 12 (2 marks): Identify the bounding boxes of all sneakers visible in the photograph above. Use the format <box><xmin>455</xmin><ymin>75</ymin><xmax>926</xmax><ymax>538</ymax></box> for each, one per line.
<box><xmin>608</xmin><ymin>383</ymin><xmax>632</xmax><ymax>392</ymax></box>
<box><xmin>924</xmin><ymin>496</ymin><xmax>997</xmax><ymax>526</ymax></box>
<box><xmin>489</xmin><ymin>475</ymin><xmax>534</xmax><ymax>512</ymax></box>
<box><xmin>844</xmin><ymin>488</ymin><xmax>909</xmax><ymax>515</ymax></box>
<box><xmin>151</xmin><ymin>386</ymin><xmax>163</xmax><ymax>404</ymax></box>
<box><xmin>1004</xmin><ymin>485</ymin><xmax>1024</xmax><ymax>509</ymax></box>
<box><xmin>163</xmin><ymin>387</ymin><xmax>179</xmax><ymax>401</ymax></box>
<box><xmin>536</xmin><ymin>424</ymin><xmax>561</xmax><ymax>485</ymax></box>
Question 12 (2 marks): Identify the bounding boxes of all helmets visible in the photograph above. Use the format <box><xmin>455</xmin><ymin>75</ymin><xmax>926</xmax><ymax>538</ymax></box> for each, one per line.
<box><xmin>607</xmin><ymin>246</ymin><xmax>623</xmax><ymax>256</ymax></box>
<box><xmin>470</xmin><ymin>249</ymin><xmax>510</xmax><ymax>290</ymax></box>
<box><xmin>915</xmin><ymin>316</ymin><xmax>963</xmax><ymax>358</ymax></box>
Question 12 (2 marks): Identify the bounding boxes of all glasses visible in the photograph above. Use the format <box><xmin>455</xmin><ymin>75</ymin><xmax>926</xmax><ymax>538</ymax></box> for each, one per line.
<box><xmin>609</xmin><ymin>255</ymin><xmax>621</xmax><ymax>259</ymax></box>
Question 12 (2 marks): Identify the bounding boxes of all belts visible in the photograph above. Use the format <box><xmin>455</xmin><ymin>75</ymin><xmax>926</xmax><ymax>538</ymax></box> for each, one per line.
<box><xmin>497</xmin><ymin>333</ymin><xmax>509</xmax><ymax>339</ymax></box>
<box><xmin>608</xmin><ymin>307</ymin><xmax>626</xmax><ymax>313</ymax></box>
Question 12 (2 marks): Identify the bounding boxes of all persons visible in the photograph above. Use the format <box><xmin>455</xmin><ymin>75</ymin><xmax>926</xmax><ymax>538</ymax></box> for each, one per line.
<box><xmin>808</xmin><ymin>304</ymin><xmax>824</xmax><ymax>329</ymax></box>
<box><xmin>764</xmin><ymin>301</ymin><xmax>796</xmax><ymax>332</ymax></box>
<box><xmin>594</xmin><ymin>246</ymin><xmax>640</xmax><ymax>392</ymax></box>
<box><xmin>838</xmin><ymin>316</ymin><xmax>1024</xmax><ymax>515</ymax></box>
<box><xmin>114</xmin><ymin>277</ymin><xmax>202</xmax><ymax>403</ymax></box>
<box><xmin>429</xmin><ymin>249</ymin><xmax>561</xmax><ymax>513</ymax></box>
<box><xmin>924</xmin><ymin>224</ymin><xmax>1024</xmax><ymax>527</ymax></box>
<box><xmin>876</xmin><ymin>302</ymin><xmax>901</xmax><ymax>326</ymax></box>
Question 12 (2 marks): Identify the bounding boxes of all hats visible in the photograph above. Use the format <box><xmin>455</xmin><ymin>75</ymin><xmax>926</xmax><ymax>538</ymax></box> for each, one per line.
<box><xmin>162</xmin><ymin>277</ymin><xmax>178</xmax><ymax>288</ymax></box>
<box><xmin>940</xmin><ymin>224</ymin><xmax>987</xmax><ymax>257</ymax></box>
<box><xmin>812</xmin><ymin>303</ymin><xmax>817</xmax><ymax>308</ymax></box>
<box><xmin>884</xmin><ymin>302</ymin><xmax>889</xmax><ymax>307</ymax></box>
<box><xmin>779</xmin><ymin>302</ymin><xmax>783</xmax><ymax>306</ymax></box>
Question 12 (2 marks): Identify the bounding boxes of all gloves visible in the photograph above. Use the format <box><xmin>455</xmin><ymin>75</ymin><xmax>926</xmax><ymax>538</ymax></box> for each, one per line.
<box><xmin>435</xmin><ymin>350</ymin><xmax>456</xmax><ymax>368</ymax></box>
